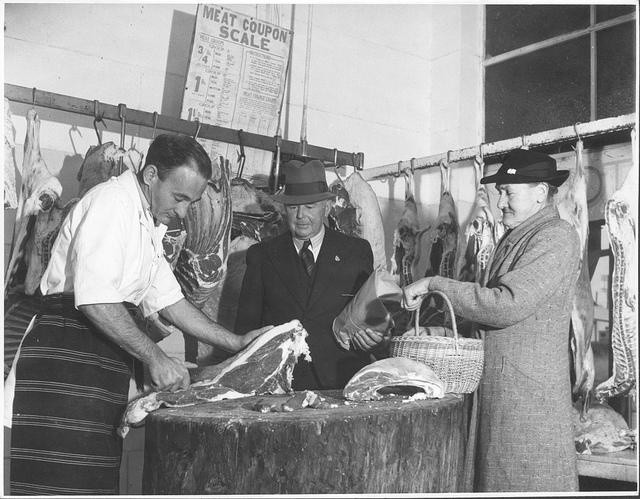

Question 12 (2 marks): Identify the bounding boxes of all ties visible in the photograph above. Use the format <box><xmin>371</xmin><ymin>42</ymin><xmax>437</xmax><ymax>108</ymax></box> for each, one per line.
<box><xmin>299</xmin><ymin>241</ymin><xmax>315</xmax><ymax>278</ymax></box>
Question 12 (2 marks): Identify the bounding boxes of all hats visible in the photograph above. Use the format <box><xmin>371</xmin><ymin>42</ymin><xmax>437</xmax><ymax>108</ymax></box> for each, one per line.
<box><xmin>480</xmin><ymin>149</ymin><xmax>570</xmax><ymax>187</ymax></box>
<box><xmin>271</xmin><ymin>159</ymin><xmax>337</xmax><ymax>205</ymax></box>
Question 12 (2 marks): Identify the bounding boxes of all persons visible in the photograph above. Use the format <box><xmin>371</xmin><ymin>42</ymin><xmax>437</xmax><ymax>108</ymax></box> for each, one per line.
<box><xmin>232</xmin><ymin>160</ymin><xmax>395</xmax><ymax>391</ymax></box>
<box><xmin>400</xmin><ymin>148</ymin><xmax>582</xmax><ymax>492</ymax></box>
<box><xmin>5</xmin><ymin>132</ymin><xmax>278</xmax><ymax>495</ymax></box>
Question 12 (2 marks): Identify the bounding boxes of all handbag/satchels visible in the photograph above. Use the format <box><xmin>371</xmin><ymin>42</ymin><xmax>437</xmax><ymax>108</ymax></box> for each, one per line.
<box><xmin>390</xmin><ymin>290</ymin><xmax>485</xmax><ymax>393</ymax></box>
